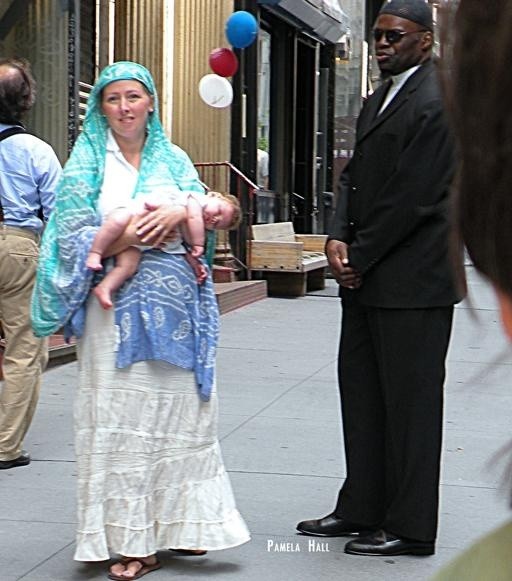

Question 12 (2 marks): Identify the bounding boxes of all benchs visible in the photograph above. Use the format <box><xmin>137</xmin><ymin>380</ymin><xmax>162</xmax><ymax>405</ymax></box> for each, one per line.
<box><xmin>245</xmin><ymin>221</ymin><xmax>330</xmax><ymax>299</ymax></box>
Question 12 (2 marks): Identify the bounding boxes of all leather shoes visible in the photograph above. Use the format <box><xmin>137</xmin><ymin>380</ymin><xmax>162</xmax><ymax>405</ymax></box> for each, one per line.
<box><xmin>344</xmin><ymin>527</ymin><xmax>434</xmax><ymax>556</ymax></box>
<box><xmin>296</xmin><ymin>512</ymin><xmax>376</xmax><ymax>536</ymax></box>
<box><xmin>0</xmin><ymin>450</ymin><xmax>30</xmax><ymax>469</ymax></box>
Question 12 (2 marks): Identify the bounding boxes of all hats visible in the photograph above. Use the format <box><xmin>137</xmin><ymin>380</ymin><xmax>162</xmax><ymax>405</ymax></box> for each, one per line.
<box><xmin>379</xmin><ymin>0</ymin><xmax>434</xmax><ymax>32</ymax></box>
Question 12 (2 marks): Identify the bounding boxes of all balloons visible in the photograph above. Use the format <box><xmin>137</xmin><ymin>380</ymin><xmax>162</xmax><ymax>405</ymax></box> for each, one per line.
<box><xmin>209</xmin><ymin>47</ymin><xmax>237</xmax><ymax>78</ymax></box>
<box><xmin>225</xmin><ymin>10</ymin><xmax>257</xmax><ymax>49</ymax></box>
<box><xmin>198</xmin><ymin>73</ymin><xmax>233</xmax><ymax>108</ymax></box>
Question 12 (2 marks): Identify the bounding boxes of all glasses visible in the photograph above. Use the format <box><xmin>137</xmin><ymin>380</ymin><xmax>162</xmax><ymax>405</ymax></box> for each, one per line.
<box><xmin>370</xmin><ymin>28</ymin><xmax>430</xmax><ymax>43</ymax></box>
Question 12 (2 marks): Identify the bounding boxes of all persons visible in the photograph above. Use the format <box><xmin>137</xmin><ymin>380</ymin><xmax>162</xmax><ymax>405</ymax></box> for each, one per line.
<box><xmin>0</xmin><ymin>56</ymin><xmax>64</xmax><ymax>469</ymax></box>
<box><xmin>437</xmin><ymin>1</ymin><xmax>512</xmax><ymax>577</ymax></box>
<box><xmin>29</xmin><ymin>60</ymin><xmax>253</xmax><ymax>580</ymax></box>
<box><xmin>84</xmin><ymin>189</ymin><xmax>244</xmax><ymax>309</ymax></box>
<box><xmin>296</xmin><ymin>1</ymin><xmax>465</xmax><ymax>556</ymax></box>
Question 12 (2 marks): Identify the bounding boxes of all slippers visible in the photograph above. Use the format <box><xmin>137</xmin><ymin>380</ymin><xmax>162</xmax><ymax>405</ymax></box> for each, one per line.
<box><xmin>169</xmin><ymin>549</ymin><xmax>207</xmax><ymax>555</ymax></box>
<box><xmin>108</xmin><ymin>554</ymin><xmax>162</xmax><ymax>581</ymax></box>
